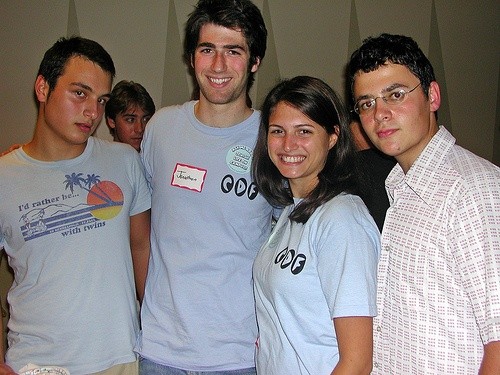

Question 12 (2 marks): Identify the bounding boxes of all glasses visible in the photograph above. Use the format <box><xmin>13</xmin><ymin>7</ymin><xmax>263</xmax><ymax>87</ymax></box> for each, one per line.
<box><xmin>350</xmin><ymin>81</ymin><xmax>426</xmax><ymax>116</ymax></box>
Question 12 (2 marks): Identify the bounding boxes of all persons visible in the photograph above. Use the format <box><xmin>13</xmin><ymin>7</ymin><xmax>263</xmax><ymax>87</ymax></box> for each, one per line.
<box><xmin>345</xmin><ymin>33</ymin><xmax>500</xmax><ymax>375</ymax></box>
<box><xmin>0</xmin><ymin>36</ymin><xmax>152</xmax><ymax>375</ymax></box>
<box><xmin>352</xmin><ymin>113</ymin><xmax>398</xmax><ymax>234</ymax></box>
<box><xmin>105</xmin><ymin>81</ymin><xmax>155</xmax><ymax>152</ymax></box>
<box><xmin>0</xmin><ymin>1</ymin><xmax>294</xmax><ymax>375</ymax></box>
<box><xmin>251</xmin><ymin>76</ymin><xmax>380</xmax><ymax>375</ymax></box>
